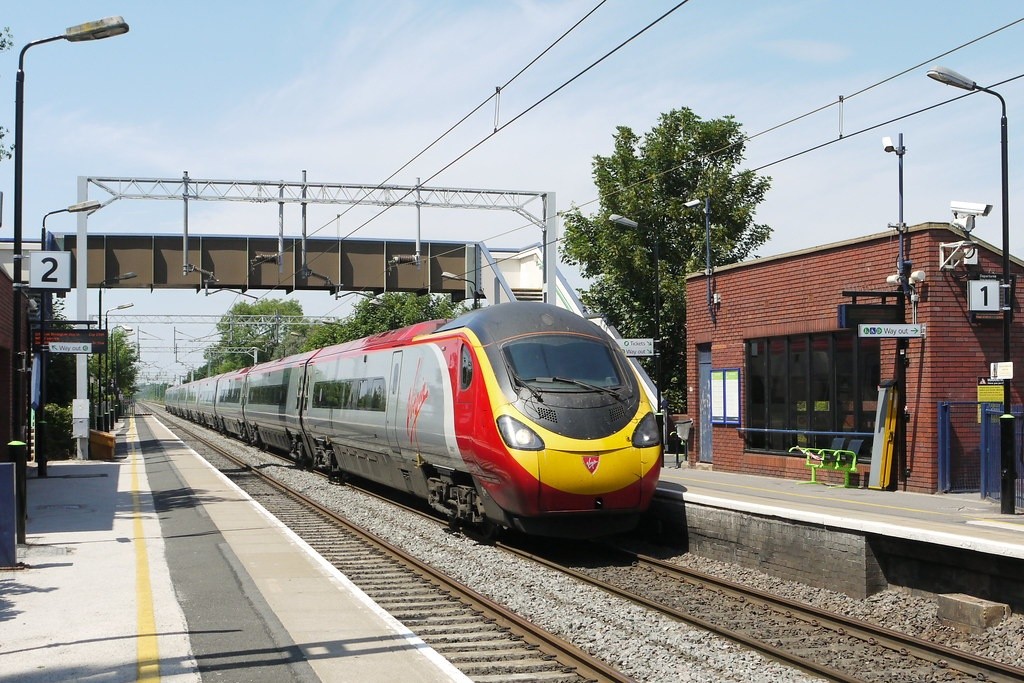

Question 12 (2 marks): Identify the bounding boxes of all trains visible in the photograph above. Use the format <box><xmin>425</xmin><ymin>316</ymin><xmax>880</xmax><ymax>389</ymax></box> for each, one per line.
<box><xmin>162</xmin><ymin>299</ymin><xmax>666</xmax><ymax>538</ymax></box>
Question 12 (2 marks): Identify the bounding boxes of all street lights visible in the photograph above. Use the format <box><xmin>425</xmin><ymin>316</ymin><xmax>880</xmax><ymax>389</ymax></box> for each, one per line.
<box><xmin>103</xmin><ymin>303</ymin><xmax>135</xmax><ymax>434</ymax></box>
<box><xmin>289</xmin><ymin>332</ymin><xmax>308</xmax><ymax>354</ymax></box>
<box><xmin>322</xmin><ymin>320</ymin><xmax>346</xmax><ymax>344</ymax></box>
<box><xmin>926</xmin><ymin>64</ymin><xmax>1017</xmax><ymax>514</ymax></box>
<box><xmin>13</xmin><ymin>16</ymin><xmax>130</xmax><ymax>551</ymax></box>
<box><xmin>609</xmin><ymin>213</ymin><xmax>664</xmax><ymax>469</ymax></box>
<box><xmin>441</xmin><ymin>270</ymin><xmax>480</xmax><ymax>310</ymax></box>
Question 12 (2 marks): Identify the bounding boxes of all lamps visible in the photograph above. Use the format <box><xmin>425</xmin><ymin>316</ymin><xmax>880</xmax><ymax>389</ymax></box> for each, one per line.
<box><xmin>886</xmin><ymin>270</ymin><xmax>926</xmax><ymax>302</ymax></box>
